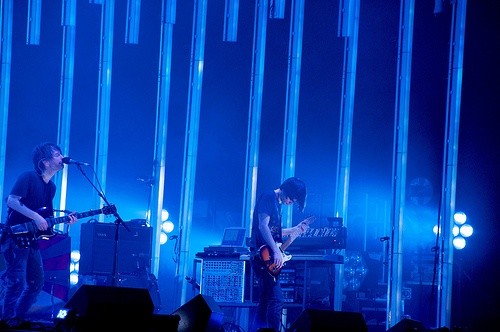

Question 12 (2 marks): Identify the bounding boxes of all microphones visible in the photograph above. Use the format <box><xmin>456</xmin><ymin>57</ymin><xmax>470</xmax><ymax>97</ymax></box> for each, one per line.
<box><xmin>62</xmin><ymin>157</ymin><xmax>90</xmax><ymax>165</ymax></box>
<box><xmin>380</xmin><ymin>236</ymin><xmax>390</xmax><ymax>241</ymax></box>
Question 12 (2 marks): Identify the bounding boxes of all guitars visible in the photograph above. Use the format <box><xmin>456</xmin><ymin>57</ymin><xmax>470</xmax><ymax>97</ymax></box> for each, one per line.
<box><xmin>8</xmin><ymin>202</ymin><xmax>117</xmax><ymax>250</ymax></box>
<box><xmin>256</xmin><ymin>215</ymin><xmax>317</xmax><ymax>276</ymax></box>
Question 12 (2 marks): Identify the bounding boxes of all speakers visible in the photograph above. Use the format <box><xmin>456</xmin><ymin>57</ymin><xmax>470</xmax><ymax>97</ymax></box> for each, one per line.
<box><xmin>387</xmin><ymin>319</ymin><xmax>427</xmax><ymax>332</ymax></box>
<box><xmin>286</xmin><ymin>308</ymin><xmax>370</xmax><ymax>332</ymax></box>
<box><xmin>28</xmin><ymin>222</ymin><xmax>225</xmax><ymax>332</ymax></box>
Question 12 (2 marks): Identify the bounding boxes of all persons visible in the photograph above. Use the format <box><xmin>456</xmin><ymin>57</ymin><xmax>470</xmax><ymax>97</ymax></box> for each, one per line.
<box><xmin>0</xmin><ymin>142</ymin><xmax>78</xmax><ymax>319</ymax></box>
<box><xmin>250</xmin><ymin>177</ymin><xmax>307</xmax><ymax>332</ymax></box>
